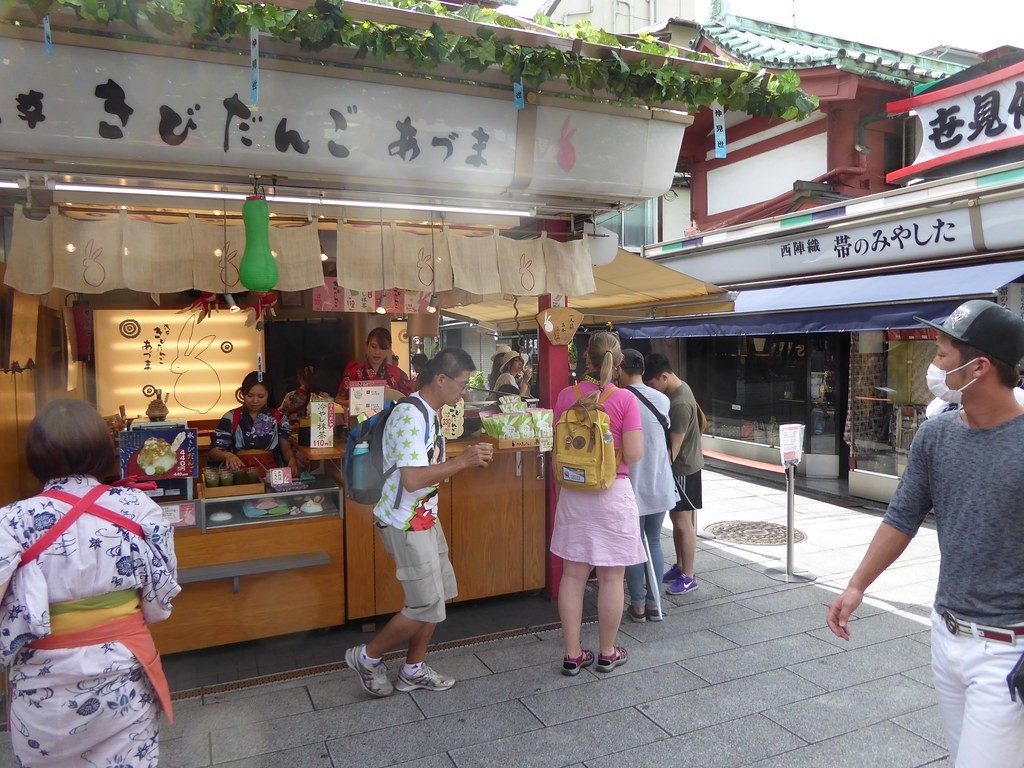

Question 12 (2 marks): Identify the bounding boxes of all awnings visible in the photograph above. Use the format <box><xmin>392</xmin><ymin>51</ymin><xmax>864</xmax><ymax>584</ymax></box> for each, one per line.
<box><xmin>607</xmin><ymin>260</ymin><xmax>1024</xmax><ymax>340</ymax></box>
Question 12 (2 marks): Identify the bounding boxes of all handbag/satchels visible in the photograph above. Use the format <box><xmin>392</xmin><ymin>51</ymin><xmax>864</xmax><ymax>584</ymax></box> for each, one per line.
<box><xmin>740</xmin><ymin>420</ymin><xmax>766</xmax><ymax>443</ymax></box>
<box><xmin>695</xmin><ymin>401</ymin><xmax>708</xmax><ymax>437</ymax></box>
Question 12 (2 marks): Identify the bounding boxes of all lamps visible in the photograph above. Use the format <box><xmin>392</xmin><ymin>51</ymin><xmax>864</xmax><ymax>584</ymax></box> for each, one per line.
<box><xmin>222</xmin><ymin>198</ymin><xmax>240</xmax><ymax>313</ymax></box>
<box><xmin>427</xmin><ymin>210</ymin><xmax>438</xmax><ymax>314</ymax></box>
<box><xmin>376</xmin><ymin>208</ymin><xmax>386</xmax><ymax>315</ymax></box>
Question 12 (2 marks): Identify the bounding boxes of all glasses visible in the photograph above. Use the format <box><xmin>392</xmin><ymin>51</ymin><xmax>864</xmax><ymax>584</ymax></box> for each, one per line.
<box><xmin>436</xmin><ymin>372</ymin><xmax>469</xmax><ymax>390</ymax></box>
<box><xmin>392</xmin><ymin>355</ymin><xmax>399</xmax><ymax>360</ymax></box>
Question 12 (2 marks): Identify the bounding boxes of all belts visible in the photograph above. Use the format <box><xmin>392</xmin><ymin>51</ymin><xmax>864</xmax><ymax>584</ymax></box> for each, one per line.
<box><xmin>933</xmin><ymin>606</ymin><xmax>1024</xmax><ymax>646</ymax></box>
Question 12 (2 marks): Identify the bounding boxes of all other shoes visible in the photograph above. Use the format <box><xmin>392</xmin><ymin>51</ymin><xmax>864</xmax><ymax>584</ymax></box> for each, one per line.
<box><xmin>822</xmin><ymin>415</ymin><xmax>831</xmax><ymax>418</ymax></box>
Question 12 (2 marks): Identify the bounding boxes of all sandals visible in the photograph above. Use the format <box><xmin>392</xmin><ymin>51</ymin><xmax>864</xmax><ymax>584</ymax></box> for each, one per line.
<box><xmin>596</xmin><ymin>646</ymin><xmax>628</xmax><ymax>673</ymax></box>
<box><xmin>561</xmin><ymin>648</ymin><xmax>595</xmax><ymax>676</ymax></box>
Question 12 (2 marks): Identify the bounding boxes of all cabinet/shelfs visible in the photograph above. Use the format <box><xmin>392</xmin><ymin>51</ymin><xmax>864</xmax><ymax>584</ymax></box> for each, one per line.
<box><xmin>331</xmin><ymin>446</ymin><xmax>545</xmax><ymax>620</ymax></box>
<box><xmin>136</xmin><ymin>479</ymin><xmax>346</xmax><ymax>652</ymax></box>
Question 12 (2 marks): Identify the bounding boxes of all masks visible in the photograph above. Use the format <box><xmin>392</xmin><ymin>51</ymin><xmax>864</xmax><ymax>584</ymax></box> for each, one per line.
<box><xmin>925</xmin><ymin>357</ymin><xmax>984</xmax><ymax>404</ymax></box>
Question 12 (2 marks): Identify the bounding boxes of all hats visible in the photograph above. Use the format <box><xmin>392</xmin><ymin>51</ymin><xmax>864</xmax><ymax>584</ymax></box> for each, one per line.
<box><xmin>912</xmin><ymin>299</ymin><xmax>1024</xmax><ymax>367</ymax></box>
<box><xmin>490</xmin><ymin>344</ymin><xmax>512</xmax><ymax>361</ymax></box>
<box><xmin>618</xmin><ymin>348</ymin><xmax>646</xmax><ymax>376</ymax></box>
<box><xmin>499</xmin><ymin>351</ymin><xmax>529</xmax><ymax>373</ymax></box>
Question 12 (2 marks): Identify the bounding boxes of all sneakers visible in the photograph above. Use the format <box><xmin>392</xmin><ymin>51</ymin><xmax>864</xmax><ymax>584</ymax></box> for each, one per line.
<box><xmin>344</xmin><ymin>643</ymin><xmax>394</xmax><ymax>698</ymax></box>
<box><xmin>663</xmin><ymin>564</ymin><xmax>683</xmax><ymax>582</ymax></box>
<box><xmin>645</xmin><ymin>603</ymin><xmax>662</xmax><ymax>621</ymax></box>
<box><xmin>626</xmin><ymin>604</ymin><xmax>647</xmax><ymax>623</ymax></box>
<box><xmin>665</xmin><ymin>573</ymin><xmax>699</xmax><ymax>595</ymax></box>
<box><xmin>394</xmin><ymin>662</ymin><xmax>457</xmax><ymax>693</ymax></box>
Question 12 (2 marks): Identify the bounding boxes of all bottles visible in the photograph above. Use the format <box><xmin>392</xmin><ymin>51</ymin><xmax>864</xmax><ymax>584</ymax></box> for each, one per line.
<box><xmin>601</xmin><ymin>423</ymin><xmax>613</xmax><ymax>444</ymax></box>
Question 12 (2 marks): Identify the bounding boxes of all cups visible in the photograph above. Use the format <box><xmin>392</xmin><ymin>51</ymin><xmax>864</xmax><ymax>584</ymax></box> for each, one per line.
<box><xmin>479</xmin><ymin>442</ymin><xmax>494</xmax><ymax>464</ymax></box>
<box><xmin>202</xmin><ymin>466</ymin><xmax>259</xmax><ymax>488</ymax></box>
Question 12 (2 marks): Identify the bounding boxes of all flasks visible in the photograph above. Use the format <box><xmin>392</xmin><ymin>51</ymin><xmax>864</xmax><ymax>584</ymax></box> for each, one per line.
<box><xmin>352</xmin><ymin>441</ymin><xmax>370</xmax><ymax>490</ymax></box>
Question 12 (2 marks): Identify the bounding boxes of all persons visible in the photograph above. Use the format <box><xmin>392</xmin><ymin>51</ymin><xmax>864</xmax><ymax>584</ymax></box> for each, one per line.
<box><xmin>334</xmin><ymin>327</ymin><xmax>541</xmax><ymax>416</ymax></box>
<box><xmin>345</xmin><ymin>348</ymin><xmax>494</xmax><ymax>697</ymax></box>
<box><xmin>0</xmin><ymin>397</ymin><xmax>178</xmax><ymax>768</ymax></box>
<box><xmin>827</xmin><ymin>300</ymin><xmax>1024</xmax><ymax>768</ymax></box>
<box><xmin>550</xmin><ymin>330</ymin><xmax>702</xmax><ymax>677</ymax></box>
<box><xmin>276</xmin><ymin>362</ymin><xmax>334</xmax><ymax>419</ymax></box>
<box><xmin>207</xmin><ymin>371</ymin><xmax>299</xmax><ymax>479</ymax></box>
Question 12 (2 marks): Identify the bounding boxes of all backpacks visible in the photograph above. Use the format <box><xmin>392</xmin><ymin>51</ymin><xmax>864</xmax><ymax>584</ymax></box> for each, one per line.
<box><xmin>343</xmin><ymin>396</ymin><xmax>430</xmax><ymax>504</ymax></box>
<box><xmin>551</xmin><ymin>384</ymin><xmax>624</xmax><ymax>494</ymax></box>
